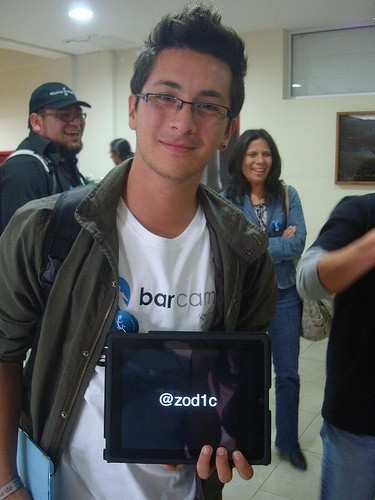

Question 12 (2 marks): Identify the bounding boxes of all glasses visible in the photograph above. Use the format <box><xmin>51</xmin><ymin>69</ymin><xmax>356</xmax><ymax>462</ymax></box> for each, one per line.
<box><xmin>136</xmin><ymin>92</ymin><xmax>233</xmax><ymax>123</ymax></box>
<box><xmin>40</xmin><ymin>112</ymin><xmax>86</xmax><ymax>122</ymax></box>
<box><xmin>108</xmin><ymin>151</ymin><xmax>113</xmax><ymax>158</ymax></box>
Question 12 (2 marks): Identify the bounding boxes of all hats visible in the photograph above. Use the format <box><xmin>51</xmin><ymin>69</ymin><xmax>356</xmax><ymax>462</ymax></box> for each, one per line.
<box><xmin>29</xmin><ymin>81</ymin><xmax>92</xmax><ymax>112</ymax></box>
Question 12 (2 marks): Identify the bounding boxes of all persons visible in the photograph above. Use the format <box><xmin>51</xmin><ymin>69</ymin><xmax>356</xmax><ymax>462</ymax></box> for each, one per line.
<box><xmin>217</xmin><ymin>129</ymin><xmax>307</xmax><ymax>470</ymax></box>
<box><xmin>111</xmin><ymin>139</ymin><xmax>134</xmax><ymax>165</ymax></box>
<box><xmin>295</xmin><ymin>191</ymin><xmax>375</xmax><ymax>500</ymax></box>
<box><xmin>0</xmin><ymin>4</ymin><xmax>278</xmax><ymax>500</ymax></box>
<box><xmin>0</xmin><ymin>82</ymin><xmax>92</xmax><ymax>235</ymax></box>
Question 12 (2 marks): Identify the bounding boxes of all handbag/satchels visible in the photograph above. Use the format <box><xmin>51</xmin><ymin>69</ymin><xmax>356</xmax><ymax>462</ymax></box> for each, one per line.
<box><xmin>302</xmin><ymin>296</ymin><xmax>332</xmax><ymax>341</ymax></box>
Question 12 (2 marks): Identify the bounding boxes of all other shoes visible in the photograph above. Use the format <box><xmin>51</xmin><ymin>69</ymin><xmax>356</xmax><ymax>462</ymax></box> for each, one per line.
<box><xmin>280</xmin><ymin>447</ymin><xmax>308</xmax><ymax>471</ymax></box>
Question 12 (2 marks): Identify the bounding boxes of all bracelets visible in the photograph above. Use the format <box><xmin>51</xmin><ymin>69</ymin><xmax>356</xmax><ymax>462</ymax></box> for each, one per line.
<box><xmin>0</xmin><ymin>476</ymin><xmax>24</xmax><ymax>500</ymax></box>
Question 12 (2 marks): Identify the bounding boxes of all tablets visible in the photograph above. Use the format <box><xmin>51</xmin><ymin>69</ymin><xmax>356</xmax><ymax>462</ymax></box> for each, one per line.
<box><xmin>103</xmin><ymin>332</ymin><xmax>271</xmax><ymax>465</ymax></box>
